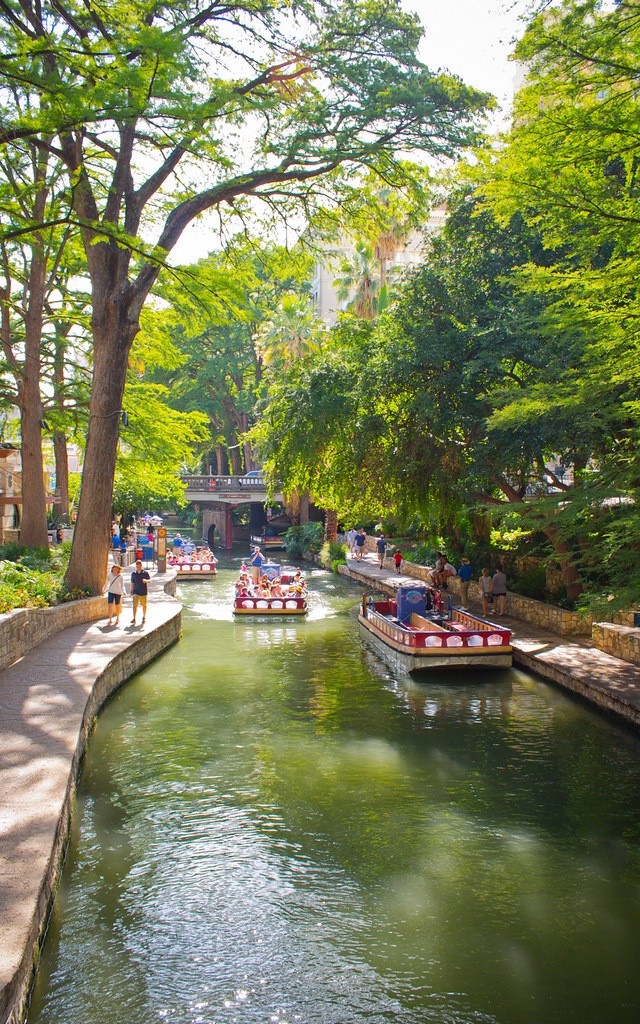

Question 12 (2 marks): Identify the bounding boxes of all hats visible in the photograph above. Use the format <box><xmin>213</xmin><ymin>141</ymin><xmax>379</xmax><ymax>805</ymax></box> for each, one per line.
<box><xmin>459</xmin><ymin>558</ymin><xmax>470</xmax><ymax>564</ymax></box>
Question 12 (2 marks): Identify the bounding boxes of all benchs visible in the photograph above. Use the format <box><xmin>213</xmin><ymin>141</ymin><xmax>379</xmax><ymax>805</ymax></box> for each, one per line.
<box><xmin>442</xmin><ymin>619</ymin><xmax>468</xmax><ymax>632</ymax></box>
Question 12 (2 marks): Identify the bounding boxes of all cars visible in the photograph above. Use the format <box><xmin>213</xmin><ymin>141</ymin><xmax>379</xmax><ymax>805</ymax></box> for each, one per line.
<box><xmin>227</xmin><ymin>470</ymin><xmax>272</xmax><ymax>490</ymax></box>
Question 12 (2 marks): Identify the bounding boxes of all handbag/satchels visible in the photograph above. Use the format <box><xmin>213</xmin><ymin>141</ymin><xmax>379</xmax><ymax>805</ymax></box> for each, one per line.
<box><xmin>485</xmin><ymin>592</ymin><xmax>494</xmax><ymax>603</ymax></box>
<box><xmin>364</xmin><ymin>547</ymin><xmax>368</xmax><ymax>555</ymax></box>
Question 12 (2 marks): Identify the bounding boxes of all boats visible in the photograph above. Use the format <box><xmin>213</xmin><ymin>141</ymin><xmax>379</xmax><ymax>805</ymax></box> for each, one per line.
<box><xmin>249</xmin><ymin>524</ymin><xmax>287</xmax><ymax>552</ymax></box>
<box><xmin>231</xmin><ymin>557</ymin><xmax>310</xmax><ymax>615</ymax></box>
<box><xmin>165</xmin><ymin>537</ymin><xmax>218</xmax><ymax>581</ymax></box>
<box><xmin>359</xmin><ymin>579</ymin><xmax>513</xmax><ymax>675</ymax></box>
<box><xmin>149</xmin><ymin>515</ymin><xmax>164</xmax><ymax>527</ymax></box>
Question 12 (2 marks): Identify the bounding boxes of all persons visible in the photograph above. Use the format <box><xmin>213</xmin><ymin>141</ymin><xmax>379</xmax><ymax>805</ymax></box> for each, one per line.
<box><xmin>147</xmin><ymin>523</ymin><xmax>155</xmax><ymax>534</ymax></box>
<box><xmin>110</xmin><ymin>521</ymin><xmax>138</xmax><ymax>554</ymax></box>
<box><xmin>428</xmin><ymin>552</ymin><xmax>457</xmax><ymax>592</ymax></box>
<box><xmin>128</xmin><ymin>560</ymin><xmax>152</xmax><ymax>624</ymax></box>
<box><xmin>58</xmin><ymin>528</ymin><xmax>65</xmax><ymax>544</ymax></box>
<box><xmin>236</xmin><ymin>546</ymin><xmax>305</xmax><ymax>597</ymax></box>
<box><xmin>347</xmin><ymin>528</ymin><xmax>368</xmax><ymax>562</ymax></box>
<box><xmin>393</xmin><ymin>549</ymin><xmax>404</xmax><ymax>575</ymax></box>
<box><xmin>102</xmin><ymin>564</ymin><xmax>127</xmax><ymax>625</ymax></box>
<box><xmin>457</xmin><ymin>558</ymin><xmax>471</xmax><ymax>611</ymax></box>
<box><xmin>166</xmin><ymin>533</ymin><xmax>214</xmax><ymax>562</ymax></box>
<box><xmin>375</xmin><ymin>535</ymin><xmax>387</xmax><ymax>569</ymax></box>
<box><xmin>479</xmin><ymin>565</ymin><xmax>506</xmax><ymax>618</ymax></box>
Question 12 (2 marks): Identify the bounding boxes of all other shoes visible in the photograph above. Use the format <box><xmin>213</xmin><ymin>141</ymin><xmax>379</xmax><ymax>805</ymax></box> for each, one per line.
<box><xmin>357</xmin><ymin>561</ymin><xmax>359</xmax><ymax>562</ymax></box>
<box><xmin>380</xmin><ymin>565</ymin><xmax>382</xmax><ymax>569</ymax></box>
<box><xmin>131</xmin><ymin>619</ymin><xmax>136</xmax><ymax>623</ymax></box>
<box><xmin>142</xmin><ymin>617</ymin><xmax>146</xmax><ymax>624</ymax></box>
<box><xmin>461</xmin><ymin>606</ymin><xmax>469</xmax><ymax>610</ymax></box>
<box><xmin>431</xmin><ymin>582</ymin><xmax>448</xmax><ymax>590</ymax></box>
<box><xmin>499</xmin><ymin>612</ymin><xmax>503</xmax><ymax>615</ymax></box>
<box><xmin>492</xmin><ymin>610</ymin><xmax>497</xmax><ymax>613</ymax></box>
